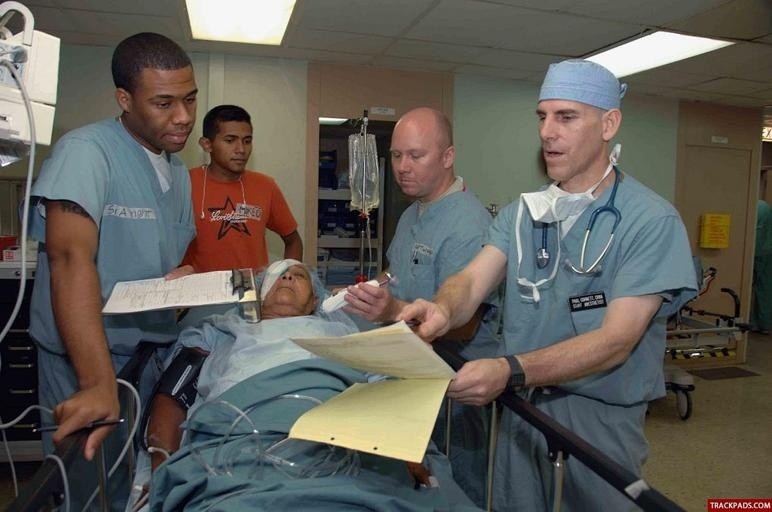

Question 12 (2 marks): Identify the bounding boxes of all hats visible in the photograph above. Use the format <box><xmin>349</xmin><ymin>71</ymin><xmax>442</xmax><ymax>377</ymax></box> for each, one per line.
<box><xmin>537</xmin><ymin>58</ymin><xmax>622</xmax><ymax>111</ymax></box>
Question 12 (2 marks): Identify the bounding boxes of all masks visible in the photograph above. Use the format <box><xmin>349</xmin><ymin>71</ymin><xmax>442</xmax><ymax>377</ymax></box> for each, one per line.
<box><xmin>520</xmin><ymin>180</ymin><xmax>603</xmax><ymax>225</ymax></box>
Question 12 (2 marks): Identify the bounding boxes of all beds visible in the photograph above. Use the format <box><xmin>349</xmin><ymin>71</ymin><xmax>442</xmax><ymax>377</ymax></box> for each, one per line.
<box><xmin>8</xmin><ymin>319</ymin><xmax>684</xmax><ymax>511</ymax></box>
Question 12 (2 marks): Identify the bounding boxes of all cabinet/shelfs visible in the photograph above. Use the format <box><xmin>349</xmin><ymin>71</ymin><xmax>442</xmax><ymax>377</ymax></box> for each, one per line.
<box><xmin>317</xmin><ymin>156</ymin><xmax>387</xmax><ymax>285</ymax></box>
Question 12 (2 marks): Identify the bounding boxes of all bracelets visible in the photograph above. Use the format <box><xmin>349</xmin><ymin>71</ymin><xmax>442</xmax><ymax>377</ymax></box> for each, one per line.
<box><xmin>503</xmin><ymin>355</ymin><xmax>528</xmax><ymax>394</ymax></box>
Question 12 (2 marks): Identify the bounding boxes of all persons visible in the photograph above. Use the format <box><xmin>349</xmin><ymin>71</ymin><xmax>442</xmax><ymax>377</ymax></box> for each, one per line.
<box><xmin>396</xmin><ymin>54</ymin><xmax>704</xmax><ymax>511</ymax></box>
<box><xmin>136</xmin><ymin>258</ymin><xmax>434</xmax><ymax>506</ymax></box>
<box><xmin>21</xmin><ymin>32</ymin><xmax>197</xmax><ymax>512</ymax></box>
<box><xmin>180</xmin><ymin>105</ymin><xmax>304</xmax><ymax>272</ymax></box>
<box><xmin>332</xmin><ymin>104</ymin><xmax>497</xmax><ymax>508</ymax></box>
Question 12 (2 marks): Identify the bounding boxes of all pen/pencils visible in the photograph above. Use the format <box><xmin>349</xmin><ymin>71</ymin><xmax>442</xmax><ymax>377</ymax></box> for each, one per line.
<box><xmin>32</xmin><ymin>418</ymin><xmax>125</xmax><ymax>433</ymax></box>
<box><xmin>373</xmin><ymin>320</ymin><xmax>422</xmax><ymax>328</ymax></box>
